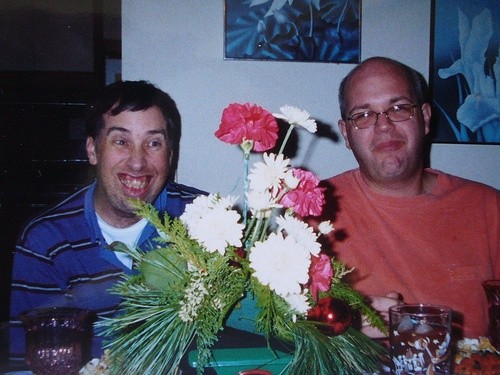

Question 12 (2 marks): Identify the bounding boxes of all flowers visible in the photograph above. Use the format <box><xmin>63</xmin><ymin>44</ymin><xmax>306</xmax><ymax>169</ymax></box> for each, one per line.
<box><xmin>93</xmin><ymin>101</ymin><xmax>392</xmax><ymax>375</ymax></box>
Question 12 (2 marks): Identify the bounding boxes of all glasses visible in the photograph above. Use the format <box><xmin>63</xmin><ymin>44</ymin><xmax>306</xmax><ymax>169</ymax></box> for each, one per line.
<box><xmin>342</xmin><ymin>104</ymin><xmax>422</xmax><ymax>130</ymax></box>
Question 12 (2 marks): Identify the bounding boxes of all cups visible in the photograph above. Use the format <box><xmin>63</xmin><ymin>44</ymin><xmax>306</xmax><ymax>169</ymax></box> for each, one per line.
<box><xmin>389</xmin><ymin>304</ymin><xmax>453</xmax><ymax>375</ymax></box>
<box><xmin>21</xmin><ymin>308</ymin><xmax>82</xmax><ymax>375</ymax></box>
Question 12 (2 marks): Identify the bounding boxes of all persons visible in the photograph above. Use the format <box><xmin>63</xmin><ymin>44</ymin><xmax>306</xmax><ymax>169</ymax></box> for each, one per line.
<box><xmin>280</xmin><ymin>57</ymin><xmax>500</xmax><ymax>342</ymax></box>
<box><xmin>6</xmin><ymin>79</ymin><xmax>220</xmax><ymax>375</ymax></box>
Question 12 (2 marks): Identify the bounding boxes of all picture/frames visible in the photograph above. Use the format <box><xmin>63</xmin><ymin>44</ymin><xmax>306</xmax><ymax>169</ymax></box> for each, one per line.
<box><xmin>429</xmin><ymin>0</ymin><xmax>500</xmax><ymax>145</ymax></box>
<box><xmin>222</xmin><ymin>0</ymin><xmax>363</xmax><ymax>64</ymax></box>
